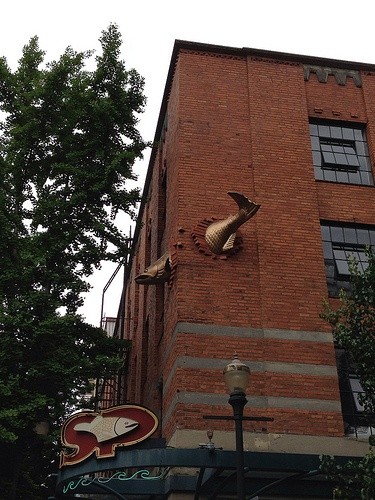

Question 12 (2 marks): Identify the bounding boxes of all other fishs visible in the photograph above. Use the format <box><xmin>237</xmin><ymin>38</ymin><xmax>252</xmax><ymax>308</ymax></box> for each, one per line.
<box><xmin>134</xmin><ymin>191</ymin><xmax>262</xmax><ymax>284</ymax></box>
<box><xmin>73</xmin><ymin>416</ymin><xmax>139</xmax><ymax>443</ymax></box>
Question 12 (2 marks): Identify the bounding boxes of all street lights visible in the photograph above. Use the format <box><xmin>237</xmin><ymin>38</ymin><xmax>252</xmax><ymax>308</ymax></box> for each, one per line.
<box><xmin>33</xmin><ymin>416</ymin><xmax>50</xmax><ymax>500</ymax></box>
<box><xmin>202</xmin><ymin>349</ymin><xmax>274</xmax><ymax>500</ymax></box>
